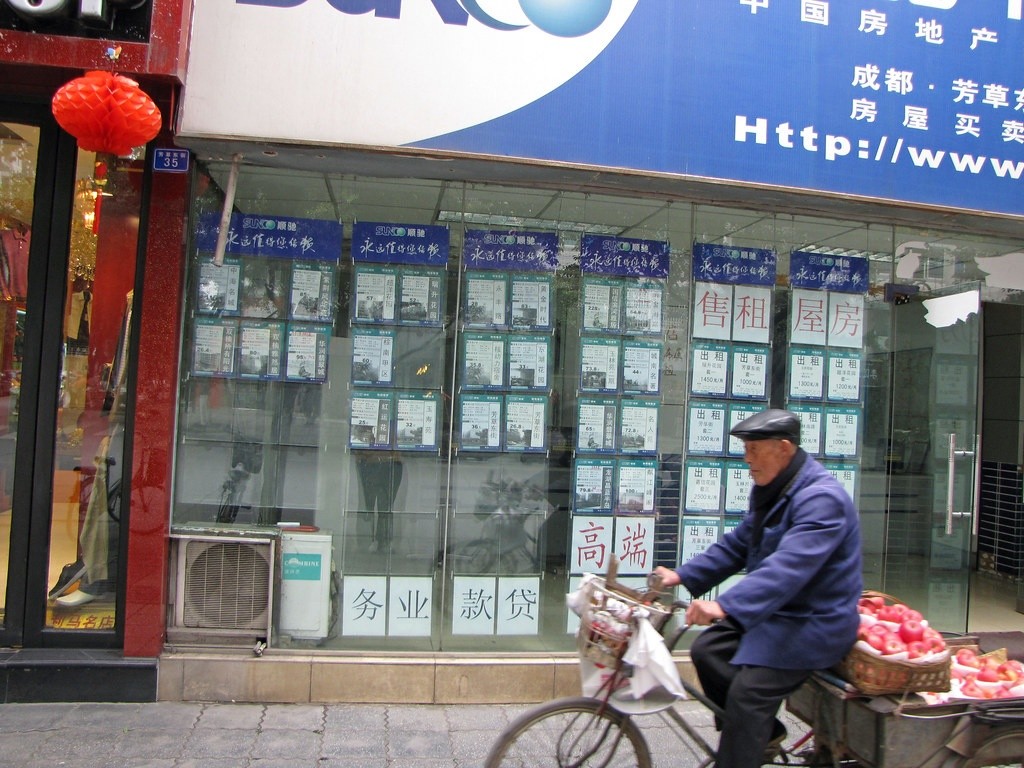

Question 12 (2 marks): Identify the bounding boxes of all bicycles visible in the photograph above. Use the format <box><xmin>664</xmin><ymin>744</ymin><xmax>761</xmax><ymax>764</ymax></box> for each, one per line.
<box><xmin>216</xmin><ymin>426</ymin><xmax>263</xmax><ymax>523</ymax></box>
<box><xmin>67</xmin><ymin>431</ymin><xmax>168</xmax><ymax>544</ymax></box>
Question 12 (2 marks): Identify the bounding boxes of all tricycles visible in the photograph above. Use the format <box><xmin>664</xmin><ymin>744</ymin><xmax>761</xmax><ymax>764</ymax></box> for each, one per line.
<box><xmin>483</xmin><ymin>572</ymin><xmax>1024</xmax><ymax>768</ymax></box>
<box><xmin>429</xmin><ymin>478</ymin><xmax>568</xmax><ymax>574</ymax></box>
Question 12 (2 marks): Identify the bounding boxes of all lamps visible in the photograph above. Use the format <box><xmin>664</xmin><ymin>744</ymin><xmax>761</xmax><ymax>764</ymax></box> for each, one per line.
<box><xmin>66</xmin><ymin>177</ymin><xmax>98</xmax><ymax>285</ymax></box>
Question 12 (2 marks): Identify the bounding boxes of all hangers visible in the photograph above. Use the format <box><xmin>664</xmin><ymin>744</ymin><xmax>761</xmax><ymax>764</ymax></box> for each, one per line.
<box><xmin>14</xmin><ymin>222</ymin><xmax>29</xmax><ymax>233</ymax></box>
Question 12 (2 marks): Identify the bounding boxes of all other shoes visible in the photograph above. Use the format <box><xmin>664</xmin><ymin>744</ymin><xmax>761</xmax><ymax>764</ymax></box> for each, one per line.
<box><xmin>765</xmin><ymin>720</ymin><xmax>788</xmax><ymax>748</ymax></box>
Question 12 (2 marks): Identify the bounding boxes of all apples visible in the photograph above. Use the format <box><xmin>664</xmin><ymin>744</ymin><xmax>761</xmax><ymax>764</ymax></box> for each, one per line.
<box><xmin>853</xmin><ymin>597</ymin><xmax>1023</xmax><ymax>696</ymax></box>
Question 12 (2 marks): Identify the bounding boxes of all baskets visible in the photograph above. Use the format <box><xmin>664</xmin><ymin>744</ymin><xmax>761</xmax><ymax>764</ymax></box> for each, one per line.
<box><xmin>231</xmin><ymin>434</ymin><xmax>263</xmax><ymax>474</ymax></box>
<box><xmin>146</xmin><ymin>447</ymin><xmax>168</xmax><ymax>487</ymax></box>
<box><xmin>977</xmin><ymin>647</ymin><xmax>1008</xmax><ymax>667</ymax></box>
<box><xmin>574</xmin><ymin>617</ymin><xmax>625</xmax><ymax>671</ymax></box>
<box><xmin>835</xmin><ymin>590</ymin><xmax>952</xmax><ymax>695</ymax></box>
<box><xmin>472</xmin><ymin>493</ymin><xmax>498</xmax><ymax>522</ymax></box>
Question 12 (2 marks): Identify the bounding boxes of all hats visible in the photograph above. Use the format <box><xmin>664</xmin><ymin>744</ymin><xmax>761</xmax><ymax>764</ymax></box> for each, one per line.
<box><xmin>729</xmin><ymin>408</ymin><xmax>802</xmax><ymax>445</ymax></box>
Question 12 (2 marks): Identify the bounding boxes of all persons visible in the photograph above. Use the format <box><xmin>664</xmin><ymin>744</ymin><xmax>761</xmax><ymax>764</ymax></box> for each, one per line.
<box><xmin>650</xmin><ymin>411</ymin><xmax>864</xmax><ymax>768</ymax></box>
<box><xmin>354</xmin><ymin>404</ymin><xmax>576</xmax><ymax>574</ymax></box>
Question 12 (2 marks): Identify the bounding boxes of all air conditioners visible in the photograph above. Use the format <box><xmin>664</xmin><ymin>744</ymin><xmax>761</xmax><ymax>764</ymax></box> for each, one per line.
<box><xmin>177</xmin><ymin>521</ymin><xmax>334</xmax><ymax>639</ymax></box>
